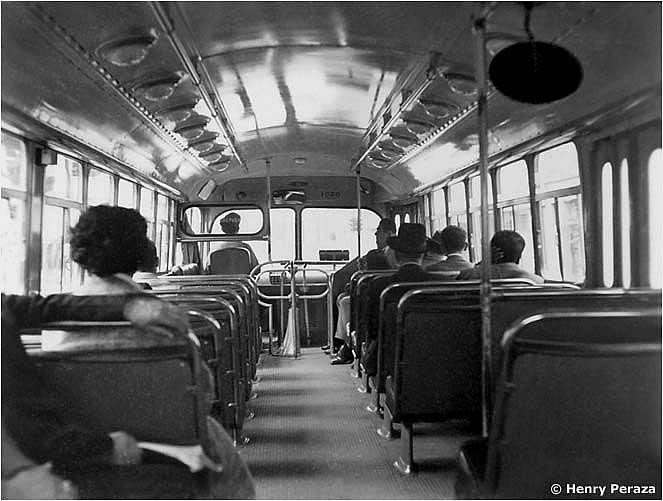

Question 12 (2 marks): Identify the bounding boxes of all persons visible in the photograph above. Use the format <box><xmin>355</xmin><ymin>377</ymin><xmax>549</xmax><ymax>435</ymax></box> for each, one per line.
<box><xmin>205</xmin><ymin>211</ymin><xmax>260</xmax><ymax>276</ymax></box>
<box><xmin>132</xmin><ymin>240</ymin><xmax>170</xmax><ymax>286</ymax></box>
<box><xmin>321</xmin><ymin>214</ymin><xmax>548</xmax><ymax>384</ymax></box>
<box><xmin>1</xmin><ymin>288</ymin><xmax>193</xmax><ymax>501</ymax></box>
<box><xmin>37</xmin><ymin>202</ymin><xmax>259</xmax><ymax>501</ymax></box>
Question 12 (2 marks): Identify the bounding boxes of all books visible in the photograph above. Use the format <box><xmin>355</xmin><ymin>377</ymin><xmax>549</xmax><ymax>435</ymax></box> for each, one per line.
<box><xmin>134</xmin><ymin>439</ymin><xmax>224</xmax><ymax>476</ymax></box>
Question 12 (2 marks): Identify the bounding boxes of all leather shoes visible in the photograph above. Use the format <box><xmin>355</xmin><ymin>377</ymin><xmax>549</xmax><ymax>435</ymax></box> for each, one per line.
<box><xmin>320</xmin><ymin>344</ymin><xmax>353</xmax><ymax>363</ymax></box>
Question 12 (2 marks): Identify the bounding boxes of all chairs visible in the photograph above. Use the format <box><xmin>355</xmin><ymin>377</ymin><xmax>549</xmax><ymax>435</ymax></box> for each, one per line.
<box><xmin>344</xmin><ymin>268</ymin><xmax>661</xmax><ymax>501</ymax></box>
<box><xmin>0</xmin><ymin>277</ymin><xmax>255</xmax><ymax>500</ymax></box>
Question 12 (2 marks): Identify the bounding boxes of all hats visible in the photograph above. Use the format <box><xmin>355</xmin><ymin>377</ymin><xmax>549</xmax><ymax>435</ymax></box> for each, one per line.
<box><xmin>385</xmin><ymin>222</ymin><xmax>440</xmax><ymax>253</ymax></box>
